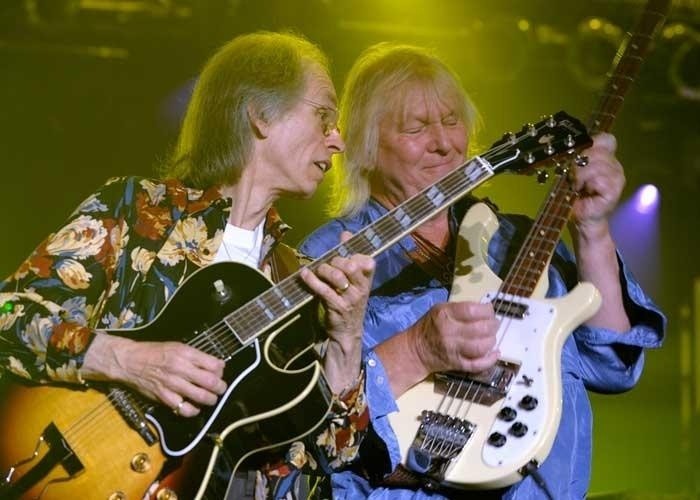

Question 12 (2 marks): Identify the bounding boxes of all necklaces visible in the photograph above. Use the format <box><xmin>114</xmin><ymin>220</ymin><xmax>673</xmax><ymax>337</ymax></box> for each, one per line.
<box><xmin>410</xmin><ymin>231</ymin><xmax>455</xmax><ymax>284</ymax></box>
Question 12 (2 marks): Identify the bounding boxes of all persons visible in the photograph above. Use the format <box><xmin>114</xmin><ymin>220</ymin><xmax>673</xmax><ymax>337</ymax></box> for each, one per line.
<box><xmin>1</xmin><ymin>32</ymin><xmax>378</xmax><ymax>499</ymax></box>
<box><xmin>297</xmin><ymin>41</ymin><xmax>668</xmax><ymax>500</ymax></box>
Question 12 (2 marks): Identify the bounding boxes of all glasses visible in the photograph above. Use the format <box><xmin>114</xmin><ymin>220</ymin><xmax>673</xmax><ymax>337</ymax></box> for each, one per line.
<box><xmin>300</xmin><ymin>97</ymin><xmax>341</xmax><ymax>132</ymax></box>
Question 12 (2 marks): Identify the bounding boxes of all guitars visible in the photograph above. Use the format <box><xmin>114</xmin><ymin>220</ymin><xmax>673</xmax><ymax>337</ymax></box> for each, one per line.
<box><xmin>388</xmin><ymin>1</ymin><xmax>675</xmax><ymax>490</ymax></box>
<box><xmin>1</xmin><ymin>110</ymin><xmax>594</xmax><ymax>496</ymax></box>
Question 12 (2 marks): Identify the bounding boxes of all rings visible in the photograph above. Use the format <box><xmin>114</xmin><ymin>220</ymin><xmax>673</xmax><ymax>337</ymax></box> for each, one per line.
<box><xmin>170</xmin><ymin>401</ymin><xmax>186</xmax><ymax>419</ymax></box>
<box><xmin>334</xmin><ymin>282</ymin><xmax>353</xmax><ymax>297</ymax></box>
